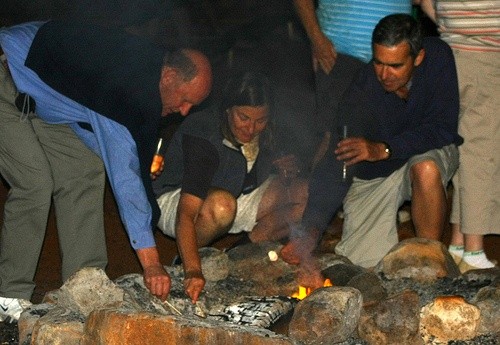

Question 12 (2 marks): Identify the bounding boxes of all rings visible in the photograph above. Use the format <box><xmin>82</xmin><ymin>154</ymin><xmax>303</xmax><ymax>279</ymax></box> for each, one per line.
<box><xmin>297</xmin><ymin>167</ymin><xmax>301</xmax><ymax>174</ymax></box>
<box><xmin>194</xmin><ymin>287</ymin><xmax>201</xmax><ymax>291</ymax></box>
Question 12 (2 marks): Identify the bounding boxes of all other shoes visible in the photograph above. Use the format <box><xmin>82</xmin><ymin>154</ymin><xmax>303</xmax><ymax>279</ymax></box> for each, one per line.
<box><xmin>399</xmin><ymin>209</ymin><xmax>411</xmax><ymax>223</ymax></box>
<box><xmin>448</xmin><ymin>250</ymin><xmax>498</xmax><ymax>268</ymax></box>
<box><xmin>172</xmin><ymin>254</ymin><xmax>182</xmax><ymax>267</ymax></box>
<box><xmin>457</xmin><ymin>259</ymin><xmax>478</xmax><ymax>273</ymax></box>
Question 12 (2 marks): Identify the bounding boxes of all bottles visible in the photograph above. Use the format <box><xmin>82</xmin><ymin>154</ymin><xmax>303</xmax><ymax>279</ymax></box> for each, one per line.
<box><xmin>339</xmin><ymin>125</ymin><xmax>351</xmax><ymax>183</ymax></box>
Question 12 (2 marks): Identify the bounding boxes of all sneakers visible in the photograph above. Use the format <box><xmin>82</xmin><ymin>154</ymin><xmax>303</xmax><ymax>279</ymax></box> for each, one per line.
<box><xmin>0</xmin><ymin>295</ymin><xmax>32</xmax><ymax>326</ymax></box>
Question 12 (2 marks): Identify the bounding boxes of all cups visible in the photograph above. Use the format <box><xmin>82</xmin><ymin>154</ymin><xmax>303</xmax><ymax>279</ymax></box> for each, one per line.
<box><xmin>151</xmin><ymin>136</ymin><xmax>168</xmax><ymax>176</ymax></box>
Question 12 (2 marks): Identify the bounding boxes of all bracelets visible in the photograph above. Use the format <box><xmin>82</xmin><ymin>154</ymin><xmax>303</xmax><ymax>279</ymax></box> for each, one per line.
<box><xmin>383</xmin><ymin>143</ymin><xmax>390</xmax><ymax>155</ymax></box>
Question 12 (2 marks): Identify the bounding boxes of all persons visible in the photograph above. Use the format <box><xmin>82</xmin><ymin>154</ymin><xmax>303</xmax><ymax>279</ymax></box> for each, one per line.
<box><xmin>151</xmin><ymin>72</ymin><xmax>309</xmax><ymax>304</ymax></box>
<box><xmin>280</xmin><ymin>14</ymin><xmax>465</xmax><ymax>269</ymax></box>
<box><xmin>0</xmin><ymin>0</ymin><xmax>500</xmax><ymax>323</ymax></box>
